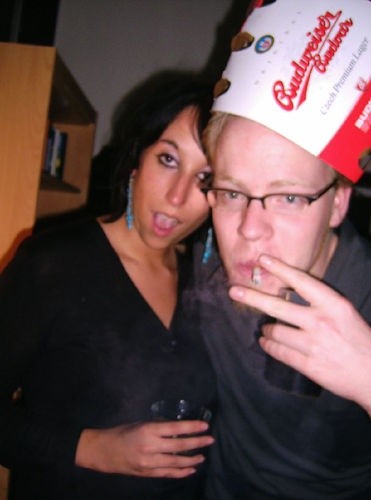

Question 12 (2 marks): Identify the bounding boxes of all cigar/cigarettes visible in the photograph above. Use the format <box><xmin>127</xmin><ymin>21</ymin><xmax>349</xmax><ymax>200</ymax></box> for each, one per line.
<box><xmin>252</xmin><ymin>267</ymin><xmax>261</xmax><ymax>285</ymax></box>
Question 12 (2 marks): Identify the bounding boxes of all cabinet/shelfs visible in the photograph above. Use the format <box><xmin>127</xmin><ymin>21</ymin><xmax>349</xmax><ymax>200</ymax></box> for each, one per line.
<box><xmin>0</xmin><ymin>41</ymin><xmax>98</xmax><ymax>276</ymax></box>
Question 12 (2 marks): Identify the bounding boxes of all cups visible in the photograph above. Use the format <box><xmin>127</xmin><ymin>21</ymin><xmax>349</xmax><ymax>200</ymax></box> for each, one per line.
<box><xmin>151</xmin><ymin>399</ymin><xmax>211</xmax><ymax>456</ymax></box>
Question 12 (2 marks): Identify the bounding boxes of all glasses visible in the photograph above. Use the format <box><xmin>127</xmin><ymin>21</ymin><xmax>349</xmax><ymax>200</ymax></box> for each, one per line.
<box><xmin>199</xmin><ymin>176</ymin><xmax>342</xmax><ymax>215</ymax></box>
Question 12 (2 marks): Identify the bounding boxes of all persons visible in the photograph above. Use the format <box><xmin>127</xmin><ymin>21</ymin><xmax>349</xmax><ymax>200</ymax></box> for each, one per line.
<box><xmin>0</xmin><ymin>0</ymin><xmax>370</xmax><ymax>500</ymax></box>
